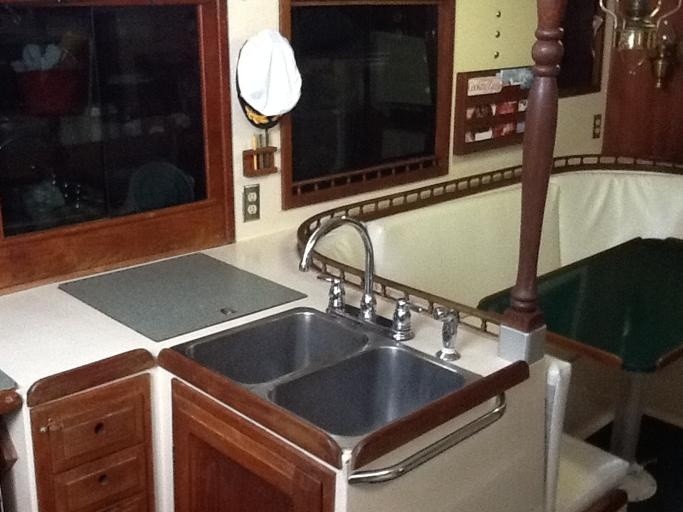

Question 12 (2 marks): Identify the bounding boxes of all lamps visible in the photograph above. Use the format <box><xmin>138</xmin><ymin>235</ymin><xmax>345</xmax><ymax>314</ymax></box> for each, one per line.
<box><xmin>599</xmin><ymin>0</ymin><xmax>682</xmax><ymax>90</ymax></box>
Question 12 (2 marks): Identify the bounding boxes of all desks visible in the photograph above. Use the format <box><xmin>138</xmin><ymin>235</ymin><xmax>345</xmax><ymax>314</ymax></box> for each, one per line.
<box><xmin>478</xmin><ymin>236</ymin><xmax>682</xmax><ymax>501</ymax></box>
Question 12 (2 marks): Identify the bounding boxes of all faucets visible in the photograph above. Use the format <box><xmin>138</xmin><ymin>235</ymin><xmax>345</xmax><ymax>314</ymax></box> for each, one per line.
<box><xmin>299</xmin><ymin>213</ymin><xmax>376</xmax><ymax>321</ymax></box>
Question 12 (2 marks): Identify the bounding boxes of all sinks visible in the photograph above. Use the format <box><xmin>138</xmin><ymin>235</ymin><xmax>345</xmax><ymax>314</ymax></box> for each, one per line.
<box><xmin>266</xmin><ymin>345</ymin><xmax>486</xmax><ymax>437</ymax></box>
<box><xmin>168</xmin><ymin>306</ymin><xmax>369</xmax><ymax>385</ymax></box>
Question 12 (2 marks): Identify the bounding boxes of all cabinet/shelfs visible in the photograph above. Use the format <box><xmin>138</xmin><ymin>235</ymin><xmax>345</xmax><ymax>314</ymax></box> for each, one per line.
<box><xmin>172</xmin><ymin>389</ymin><xmax>337</xmax><ymax>512</ymax></box>
<box><xmin>28</xmin><ymin>372</ymin><xmax>156</xmax><ymax>512</ymax></box>
<box><xmin>453</xmin><ymin>65</ymin><xmax>533</xmax><ymax>156</ymax></box>
